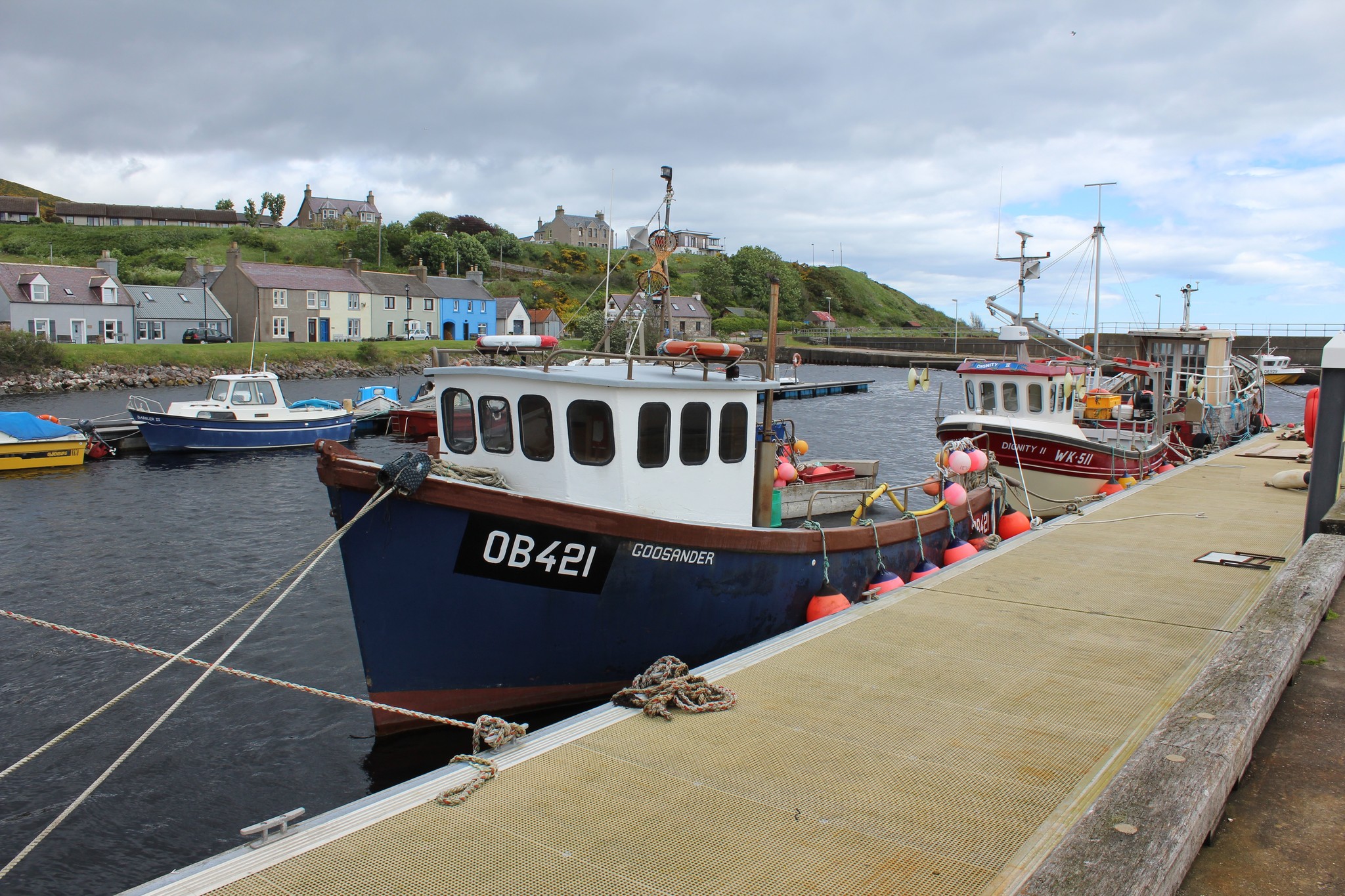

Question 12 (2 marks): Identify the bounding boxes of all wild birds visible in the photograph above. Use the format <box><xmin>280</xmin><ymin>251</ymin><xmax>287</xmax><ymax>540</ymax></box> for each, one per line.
<box><xmin>1069</xmin><ymin>31</ymin><xmax>1076</xmax><ymax>36</ymax></box>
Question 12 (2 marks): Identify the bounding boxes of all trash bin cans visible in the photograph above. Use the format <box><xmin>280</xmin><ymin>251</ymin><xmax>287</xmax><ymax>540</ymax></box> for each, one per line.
<box><xmin>96</xmin><ymin>335</ymin><xmax>104</xmax><ymax>344</ymax></box>
<box><xmin>288</xmin><ymin>331</ymin><xmax>295</xmax><ymax>341</ymax></box>
<box><xmin>771</xmin><ymin>490</ymin><xmax>783</xmax><ymax>526</ymax></box>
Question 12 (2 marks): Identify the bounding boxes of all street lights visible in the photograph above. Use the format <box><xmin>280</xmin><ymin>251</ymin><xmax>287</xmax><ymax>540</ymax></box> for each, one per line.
<box><xmin>831</xmin><ymin>249</ymin><xmax>834</xmax><ymax>267</ymax></box>
<box><xmin>723</xmin><ymin>237</ymin><xmax>726</xmax><ymax>254</ymax></box>
<box><xmin>951</xmin><ymin>299</ymin><xmax>958</xmax><ymax>354</ymax></box>
<box><xmin>721</xmin><ymin>246</ymin><xmax>724</xmax><ymax>262</ymax></box>
<box><xmin>811</xmin><ymin>244</ymin><xmax>814</xmax><ymax>266</ymax></box>
<box><xmin>404</xmin><ymin>283</ymin><xmax>411</xmax><ymax>340</ymax></box>
<box><xmin>262</xmin><ymin>249</ymin><xmax>266</xmax><ymax>263</ymax></box>
<box><xmin>825</xmin><ymin>297</ymin><xmax>832</xmax><ymax>345</ymax></box>
<box><xmin>200</xmin><ymin>274</ymin><xmax>208</xmax><ymax>344</ymax></box>
<box><xmin>48</xmin><ymin>242</ymin><xmax>53</xmax><ymax>265</ymax></box>
<box><xmin>1155</xmin><ymin>294</ymin><xmax>1161</xmax><ymax>329</ymax></box>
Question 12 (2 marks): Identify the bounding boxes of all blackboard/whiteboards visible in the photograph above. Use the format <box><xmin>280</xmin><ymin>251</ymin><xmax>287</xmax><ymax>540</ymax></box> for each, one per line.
<box><xmin>1193</xmin><ymin>550</ymin><xmax>1254</xmax><ymax>566</ymax></box>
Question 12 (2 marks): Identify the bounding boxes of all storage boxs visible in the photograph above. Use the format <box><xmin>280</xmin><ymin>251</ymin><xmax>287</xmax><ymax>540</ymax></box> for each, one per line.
<box><xmin>1073</xmin><ymin>395</ymin><xmax>1134</xmax><ymax>422</ymax></box>
<box><xmin>799</xmin><ymin>463</ymin><xmax>855</xmax><ymax>483</ymax></box>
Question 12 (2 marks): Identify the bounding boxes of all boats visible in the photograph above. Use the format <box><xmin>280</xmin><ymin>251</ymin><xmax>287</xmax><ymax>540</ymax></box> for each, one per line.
<box><xmin>311</xmin><ymin>164</ymin><xmax>1007</xmax><ymax>744</ymax></box>
<box><xmin>217</xmin><ymin>384</ymin><xmax>510</xmax><ymax>444</ymax></box>
<box><xmin>0</xmin><ymin>410</ymin><xmax>149</xmax><ymax>473</ymax></box>
<box><xmin>125</xmin><ymin>318</ymin><xmax>355</xmax><ymax>458</ymax></box>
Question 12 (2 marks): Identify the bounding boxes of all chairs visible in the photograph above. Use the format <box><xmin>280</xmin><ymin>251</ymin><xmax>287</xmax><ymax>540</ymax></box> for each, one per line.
<box><xmin>334</xmin><ymin>334</ymin><xmax>347</xmax><ymax>342</ymax></box>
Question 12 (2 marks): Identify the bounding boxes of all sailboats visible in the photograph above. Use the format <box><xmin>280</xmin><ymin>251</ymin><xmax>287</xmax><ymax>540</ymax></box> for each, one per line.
<box><xmin>942</xmin><ymin>178</ymin><xmax>1310</xmax><ymax>525</ymax></box>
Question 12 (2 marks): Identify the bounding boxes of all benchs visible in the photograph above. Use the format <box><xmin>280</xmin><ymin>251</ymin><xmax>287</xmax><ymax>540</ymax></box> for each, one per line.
<box><xmin>87</xmin><ymin>335</ymin><xmax>105</xmax><ymax>344</ymax></box>
<box><xmin>470</xmin><ymin>333</ymin><xmax>481</xmax><ymax>340</ymax></box>
<box><xmin>389</xmin><ymin>335</ymin><xmax>397</xmax><ymax>341</ymax></box>
<box><xmin>57</xmin><ymin>335</ymin><xmax>76</xmax><ymax>344</ymax></box>
<box><xmin>431</xmin><ymin>335</ymin><xmax>440</xmax><ymax>340</ymax></box>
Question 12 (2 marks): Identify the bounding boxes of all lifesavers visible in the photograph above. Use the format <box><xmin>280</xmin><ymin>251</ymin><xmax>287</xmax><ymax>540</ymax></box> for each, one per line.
<box><xmin>655</xmin><ymin>340</ymin><xmax>746</xmax><ymax>358</ymax></box>
<box><xmin>1261</xmin><ymin>353</ymin><xmax>1268</xmax><ymax>355</ymax></box>
<box><xmin>1126</xmin><ymin>390</ymin><xmax>1154</xmax><ymax>409</ymax></box>
<box><xmin>1180</xmin><ymin>325</ymin><xmax>1207</xmax><ymax>330</ymax></box>
<box><xmin>1082</xmin><ymin>388</ymin><xmax>1112</xmax><ymax>404</ymax></box>
<box><xmin>1250</xmin><ymin>414</ymin><xmax>1261</xmax><ymax>435</ymax></box>
<box><xmin>456</xmin><ymin>359</ymin><xmax>472</xmax><ymax>366</ymax></box>
<box><xmin>1192</xmin><ymin>433</ymin><xmax>1212</xmax><ymax>457</ymax></box>
<box><xmin>36</xmin><ymin>414</ymin><xmax>61</xmax><ymax>425</ymax></box>
<box><xmin>476</xmin><ymin>334</ymin><xmax>559</xmax><ymax>349</ymax></box>
<box><xmin>792</xmin><ymin>353</ymin><xmax>802</xmax><ymax>367</ymax></box>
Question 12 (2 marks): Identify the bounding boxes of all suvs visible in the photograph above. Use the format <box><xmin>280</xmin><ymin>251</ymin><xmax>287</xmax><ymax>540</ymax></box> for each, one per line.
<box><xmin>182</xmin><ymin>327</ymin><xmax>234</xmax><ymax>344</ymax></box>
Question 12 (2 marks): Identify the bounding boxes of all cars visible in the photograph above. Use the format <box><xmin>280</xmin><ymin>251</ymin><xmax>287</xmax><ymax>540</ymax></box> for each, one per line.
<box><xmin>434</xmin><ymin>232</ymin><xmax>449</xmax><ymax>239</ymax></box>
<box><xmin>396</xmin><ymin>329</ymin><xmax>429</xmax><ymax>341</ymax></box>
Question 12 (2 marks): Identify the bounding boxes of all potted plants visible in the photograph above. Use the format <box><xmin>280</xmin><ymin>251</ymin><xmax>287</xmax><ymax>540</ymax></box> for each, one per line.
<box><xmin>348</xmin><ymin>338</ymin><xmax>351</xmax><ymax>342</ymax></box>
<box><xmin>362</xmin><ymin>338</ymin><xmax>368</xmax><ymax>342</ymax></box>
<box><xmin>367</xmin><ymin>337</ymin><xmax>375</xmax><ymax>342</ymax></box>
